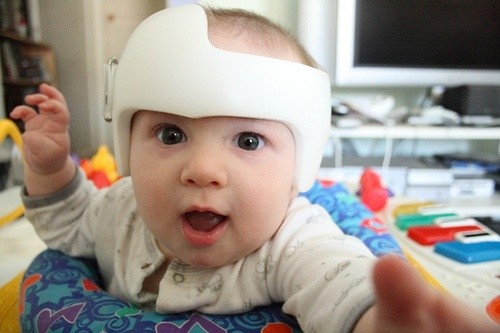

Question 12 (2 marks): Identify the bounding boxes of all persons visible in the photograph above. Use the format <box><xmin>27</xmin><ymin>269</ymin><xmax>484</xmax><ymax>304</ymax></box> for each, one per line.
<box><xmin>10</xmin><ymin>2</ymin><xmax>499</xmax><ymax>333</ymax></box>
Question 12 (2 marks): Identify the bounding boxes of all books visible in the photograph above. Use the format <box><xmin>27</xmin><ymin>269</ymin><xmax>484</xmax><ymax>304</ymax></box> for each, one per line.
<box><xmin>0</xmin><ymin>0</ymin><xmax>54</xmax><ymax>139</ymax></box>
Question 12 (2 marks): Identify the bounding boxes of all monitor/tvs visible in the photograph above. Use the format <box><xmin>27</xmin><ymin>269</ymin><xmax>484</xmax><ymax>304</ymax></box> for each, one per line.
<box><xmin>334</xmin><ymin>1</ymin><xmax>500</xmax><ymax>86</ymax></box>
